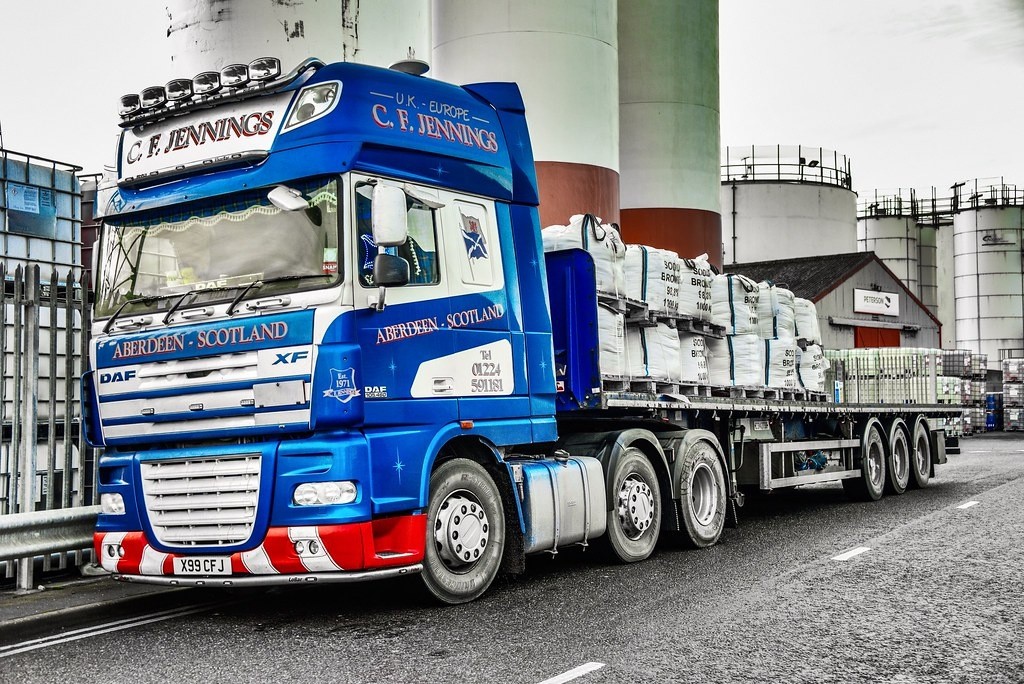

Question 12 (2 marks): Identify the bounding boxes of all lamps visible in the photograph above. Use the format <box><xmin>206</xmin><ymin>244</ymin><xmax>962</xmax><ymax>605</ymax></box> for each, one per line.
<box><xmin>800</xmin><ymin>158</ymin><xmax>818</xmax><ymax>167</ymax></box>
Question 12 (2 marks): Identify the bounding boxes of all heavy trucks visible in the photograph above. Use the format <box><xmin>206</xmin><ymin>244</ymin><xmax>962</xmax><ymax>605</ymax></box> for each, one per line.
<box><xmin>79</xmin><ymin>56</ymin><xmax>965</xmax><ymax>607</ymax></box>
